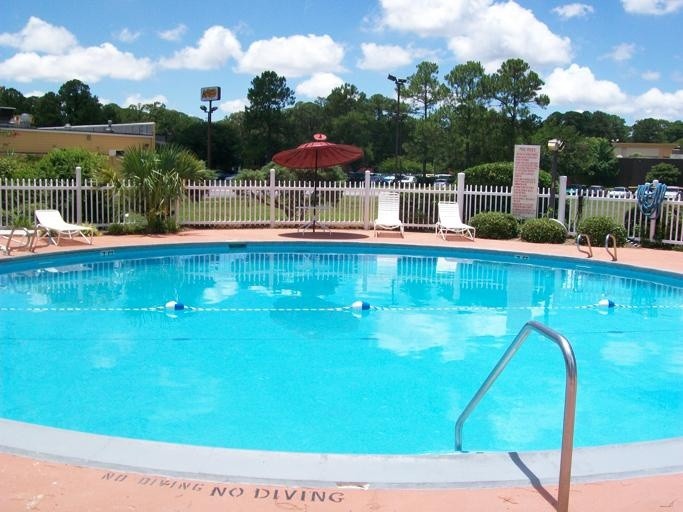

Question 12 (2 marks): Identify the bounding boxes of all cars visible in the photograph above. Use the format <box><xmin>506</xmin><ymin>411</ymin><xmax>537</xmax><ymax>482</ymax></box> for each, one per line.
<box><xmin>342</xmin><ymin>172</ymin><xmax>454</xmax><ymax>189</ymax></box>
<box><xmin>216</xmin><ymin>168</ymin><xmax>240</xmax><ymax>181</ymax></box>
<box><xmin>568</xmin><ymin>184</ymin><xmax>682</xmax><ymax>206</ymax></box>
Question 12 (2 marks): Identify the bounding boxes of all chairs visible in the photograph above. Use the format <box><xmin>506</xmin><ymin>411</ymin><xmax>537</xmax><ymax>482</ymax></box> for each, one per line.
<box><xmin>434</xmin><ymin>200</ymin><xmax>477</xmax><ymax>245</ymax></box>
<box><xmin>0</xmin><ymin>223</ymin><xmax>29</xmax><ymax>256</ymax></box>
<box><xmin>371</xmin><ymin>190</ymin><xmax>406</xmax><ymax>238</ymax></box>
<box><xmin>33</xmin><ymin>209</ymin><xmax>93</xmax><ymax>248</ymax></box>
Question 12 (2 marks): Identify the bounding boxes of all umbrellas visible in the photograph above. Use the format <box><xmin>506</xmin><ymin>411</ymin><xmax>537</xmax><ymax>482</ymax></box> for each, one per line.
<box><xmin>273</xmin><ymin>141</ymin><xmax>363</xmax><ymax>233</ymax></box>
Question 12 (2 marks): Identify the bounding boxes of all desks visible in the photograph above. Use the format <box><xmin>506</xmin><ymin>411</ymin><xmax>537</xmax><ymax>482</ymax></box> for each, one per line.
<box><xmin>294</xmin><ymin>203</ymin><xmax>333</xmax><ymax>236</ymax></box>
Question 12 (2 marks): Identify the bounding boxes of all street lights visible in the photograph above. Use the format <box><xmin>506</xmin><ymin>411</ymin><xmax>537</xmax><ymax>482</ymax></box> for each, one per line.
<box><xmin>387</xmin><ymin>73</ymin><xmax>406</xmax><ymax>191</ymax></box>
<box><xmin>199</xmin><ymin>104</ymin><xmax>217</xmax><ymax>182</ymax></box>
<box><xmin>544</xmin><ymin>137</ymin><xmax>563</xmax><ymax>219</ymax></box>
